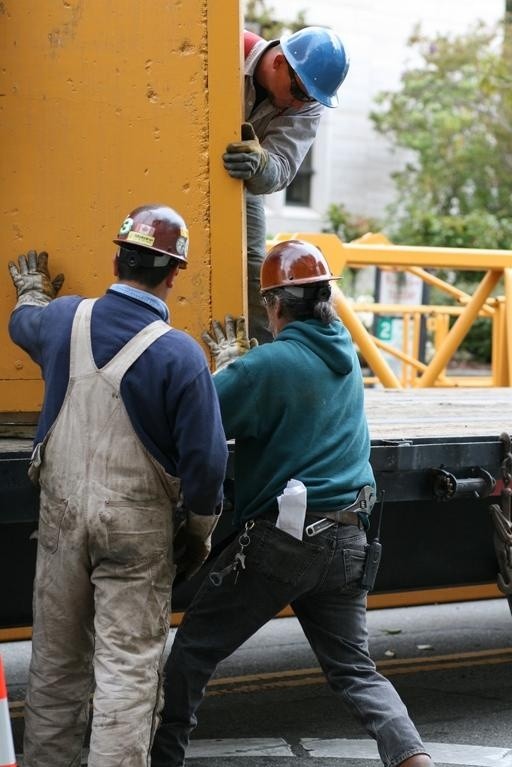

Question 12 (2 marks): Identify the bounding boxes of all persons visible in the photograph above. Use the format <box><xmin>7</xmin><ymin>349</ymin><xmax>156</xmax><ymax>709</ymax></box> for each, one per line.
<box><xmin>6</xmin><ymin>199</ymin><xmax>230</xmax><ymax>767</ymax></box>
<box><xmin>149</xmin><ymin>241</ymin><xmax>437</xmax><ymax>766</ymax></box>
<box><xmin>220</xmin><ymin>23</ymin><xmax>350</xmax><ymax>351</ymax></box>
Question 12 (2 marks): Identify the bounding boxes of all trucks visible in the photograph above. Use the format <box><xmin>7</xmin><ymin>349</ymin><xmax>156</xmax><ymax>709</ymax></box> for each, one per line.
<box><xmin>2</xmin><ymin>389</ymin><xmax>509</xmax><ymax>646</ymax></box>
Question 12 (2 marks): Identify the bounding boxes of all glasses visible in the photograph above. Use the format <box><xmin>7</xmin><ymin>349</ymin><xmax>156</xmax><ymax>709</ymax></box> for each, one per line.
<box><xmin>283</xmin><ymin>56</ymin><xmax>316</xmax><ymax>102</ymax></box>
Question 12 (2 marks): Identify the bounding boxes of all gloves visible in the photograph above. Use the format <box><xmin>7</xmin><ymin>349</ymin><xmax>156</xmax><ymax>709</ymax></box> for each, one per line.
<box><xmin>223</xmin><ymin>122</ymin><xmax>278</xmax><ymax>195</ymax></box>
<box><xmin>202</xmin><ymin>314</ymin><xmax>259</xmax><ymax>370</ymax></box>
<box><xmin>173</xmin><ymin>501</ymin><xmax>224</xmax><ymax>581</ymax></box>
<box><xmin>8</xmin><ymin>249</ymin><xmax>64</xmax><ymax>320</ymax></box>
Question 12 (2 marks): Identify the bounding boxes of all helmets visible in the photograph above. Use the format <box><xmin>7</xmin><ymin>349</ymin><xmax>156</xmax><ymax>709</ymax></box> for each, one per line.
<box><xmin>260</xmin><ymin>240</ymin><xmax>344</xmax><ymax>295</ymax></box>
<box><xmin>280</xmin><ymin>26</ymin><xmax>350</xmax><ymax>108</ymax></box>
<box><xmin>112</xmin><ymin>204</ymin><xmax>190</xmax><ymax>269</ymax></box>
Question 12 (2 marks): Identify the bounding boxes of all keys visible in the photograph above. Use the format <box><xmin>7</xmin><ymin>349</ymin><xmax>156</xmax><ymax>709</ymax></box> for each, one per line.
<box><xmin>232</xmin><ymin>551</ymin><xmax>247</xmax><ymax>585</ymax></box>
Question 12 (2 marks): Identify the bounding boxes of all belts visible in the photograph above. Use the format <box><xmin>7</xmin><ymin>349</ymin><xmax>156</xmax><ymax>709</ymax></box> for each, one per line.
<box><xmin>306</xmin><ymin>510</ymin><xmax>359</xmax><ymax>525</ymax></box>
